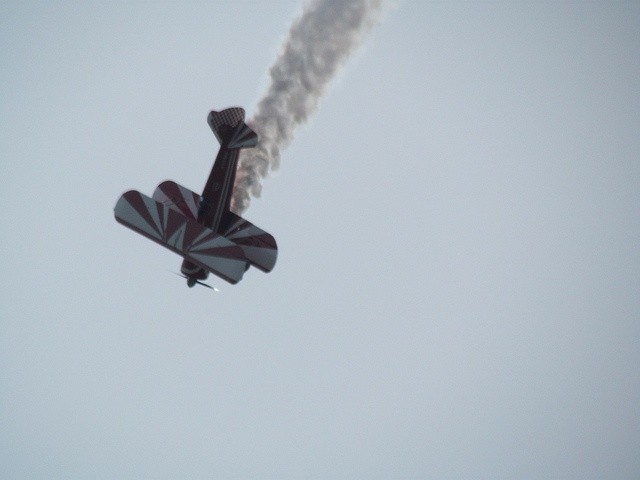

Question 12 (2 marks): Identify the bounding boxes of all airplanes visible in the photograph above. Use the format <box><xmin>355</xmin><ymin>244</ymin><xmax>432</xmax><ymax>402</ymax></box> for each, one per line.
<box><xmin>113</xmin><ymin>106</ymin><xmax>278</xmax><ymax>293</ymax></box>
